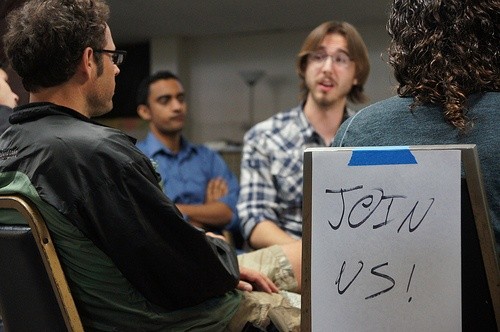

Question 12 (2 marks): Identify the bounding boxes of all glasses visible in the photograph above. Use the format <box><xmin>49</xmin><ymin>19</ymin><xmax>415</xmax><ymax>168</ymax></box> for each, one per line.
<box><xmin>305</xmin><ymin>51</ymin><xmax>353</xmax><ymax>68</ymax></box>
<box><xmin>93</xmin><ymin>49</ymin><xmax>127</xmax><ymax>65</ymax></box>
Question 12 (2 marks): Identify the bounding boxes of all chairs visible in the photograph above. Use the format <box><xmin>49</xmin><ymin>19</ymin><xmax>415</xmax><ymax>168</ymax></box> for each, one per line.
<box><xmin>0</xmin><ymin>196</ymin><xmax>85</xmax><ymax>332</ymax></box>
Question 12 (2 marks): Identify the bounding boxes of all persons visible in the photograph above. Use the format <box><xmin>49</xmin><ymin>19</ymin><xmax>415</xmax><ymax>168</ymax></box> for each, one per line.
<box><xmin>236</xmin><ymin>20</ymin><xmax>370</xmax><ymax>251</ymax></box>
<box><xmin>329</xmin><ymin>0</ymin><xmax>500</xmax><ymax>331</ymax></box>
<box><xmin>0</xmin><ymin>69</ymin><xmax>20</xmax><ymax>139</ymax></box>
<box><xmin>0</xmin><ymin>0</ymin><xmax>303</xmax><ymax>332</ymax></box>
<box><xmin>125</xmin><ymin>69</ymin><xmax>239</xmax><ymax>249</ymax></box>
